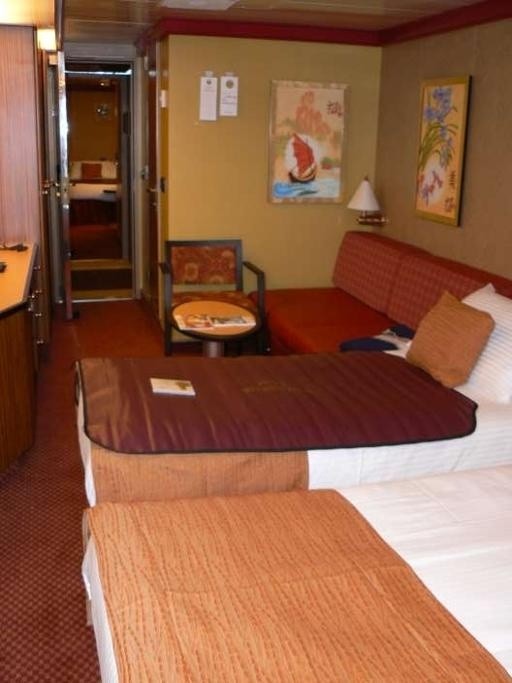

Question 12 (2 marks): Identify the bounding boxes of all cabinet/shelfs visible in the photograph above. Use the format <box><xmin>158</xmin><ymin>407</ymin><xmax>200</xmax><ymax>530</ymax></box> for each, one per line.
<box><xmin>1</xmin><ymin>251</ymin><xmax>44</xmax><ymax>474</ymax></box>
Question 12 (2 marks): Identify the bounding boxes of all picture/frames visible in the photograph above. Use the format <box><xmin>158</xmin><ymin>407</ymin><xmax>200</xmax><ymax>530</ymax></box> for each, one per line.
<box><xmin>266</xmin><ymin>78</ymin><xmax>351</xmax><ymax>206</ymax></box>
<box><xmin>412</xmin><ymin>74</ymin><xmax>471</xmax><ymax>227</ymax></box>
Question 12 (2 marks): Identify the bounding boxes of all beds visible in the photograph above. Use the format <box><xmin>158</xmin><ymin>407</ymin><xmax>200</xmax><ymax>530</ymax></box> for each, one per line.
<box><xmin>69</xmin><ymin>160</ymin><xmax>118</xmax><ymax>225</ymax></box>
<box><xmin>75</xmin><ymin>463</ymin><xmax>511</xmax><ymax>682</ymax></box>
<box><xmin>71</xmin><ymin>351</ymin><xmax>509</xmax><ymax>505</ymax></box>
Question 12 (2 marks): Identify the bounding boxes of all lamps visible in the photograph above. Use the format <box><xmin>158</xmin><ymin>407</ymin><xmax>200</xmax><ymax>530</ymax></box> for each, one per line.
<box><xmin>346</xmin><ymin>175</ymin><xmax>388</xmax><ymax>229</ymax></box>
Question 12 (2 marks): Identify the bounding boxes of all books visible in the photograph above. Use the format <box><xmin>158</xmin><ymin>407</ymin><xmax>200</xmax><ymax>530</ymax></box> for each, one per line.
<box><xmin>174</xmin><ymin>313</ymin><xmax>256</xmax><ymax>331</ymax></box>
<box><xmin>150</xmin><ymin>376</ymin><xmax>196</xmax><ymax>396</ymax></box>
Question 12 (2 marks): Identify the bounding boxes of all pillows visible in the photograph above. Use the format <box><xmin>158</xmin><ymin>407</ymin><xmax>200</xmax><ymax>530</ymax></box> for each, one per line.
<box><xmin>403</xmin><ymin>280</ymin><xmax>512</xmax><ymax>403</ymax></box>
<box><xmin>83</xmin><ymin>161</ymin><xmax>102</xmax><ymax>180</ymax></box>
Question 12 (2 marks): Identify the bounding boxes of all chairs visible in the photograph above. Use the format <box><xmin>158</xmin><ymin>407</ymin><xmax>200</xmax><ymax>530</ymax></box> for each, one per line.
<box><xmin>158</xmin><ymin>238</ymin><xmax>269</xmax><ymax>357</ymax></box>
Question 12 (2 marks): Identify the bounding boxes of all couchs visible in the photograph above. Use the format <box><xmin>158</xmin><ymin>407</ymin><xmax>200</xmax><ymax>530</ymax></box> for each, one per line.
<box><xmin>248</xmin><ymin>228</ymin><xmax>504</xmax><ymax>354</ymax></box>
